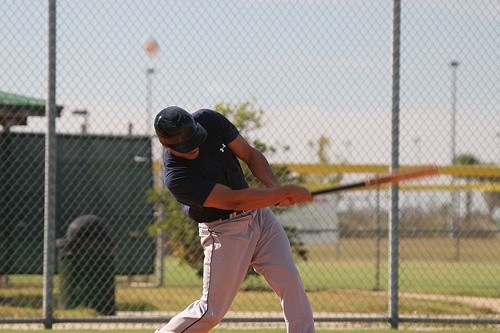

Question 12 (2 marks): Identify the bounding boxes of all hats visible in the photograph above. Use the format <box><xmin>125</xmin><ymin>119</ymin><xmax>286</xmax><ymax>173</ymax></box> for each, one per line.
<box><xmin>154</xmin><ymin>105</ymin><xmax>207</xmax><ymax>153</ymax></box>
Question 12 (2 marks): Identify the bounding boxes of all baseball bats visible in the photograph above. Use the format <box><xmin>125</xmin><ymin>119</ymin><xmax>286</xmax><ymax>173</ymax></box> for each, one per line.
<box><xmin>274</xmin><ymin>167</ymin><xmax>440</xmax><ymax>206</ymax></box>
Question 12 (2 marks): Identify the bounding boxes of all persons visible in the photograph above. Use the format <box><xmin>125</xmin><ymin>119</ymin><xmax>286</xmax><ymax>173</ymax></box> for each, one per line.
<box><xmin>154</xmin><ymin>106</ymin><xmax>316</xmax><ymax>333</ymax></box>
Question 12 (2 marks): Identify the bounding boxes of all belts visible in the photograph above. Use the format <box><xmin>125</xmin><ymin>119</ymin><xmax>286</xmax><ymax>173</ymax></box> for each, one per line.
<box><xmin>221</xmin><ymin>208</ymin><xmax>254</xmax><ymax>221</ymax></box>
